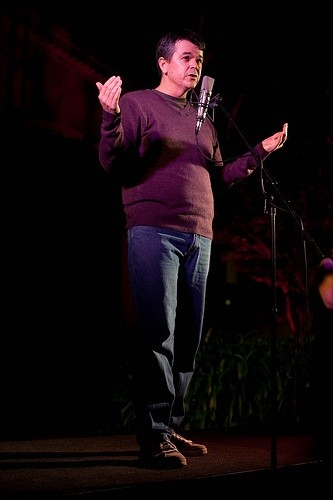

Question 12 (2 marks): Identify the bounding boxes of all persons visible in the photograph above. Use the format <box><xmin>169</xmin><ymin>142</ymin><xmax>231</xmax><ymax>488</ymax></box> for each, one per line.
<box><xmin>96</xmin><ymin>27</ymin><xmax>288</xmax><ymax>471</ymax></box>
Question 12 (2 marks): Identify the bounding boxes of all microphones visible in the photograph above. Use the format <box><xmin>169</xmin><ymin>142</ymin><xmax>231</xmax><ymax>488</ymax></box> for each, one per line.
<box><xmin>195</xmin><ymin>75</ymin><xmax>215</xmax><ymax>137</ymax></box>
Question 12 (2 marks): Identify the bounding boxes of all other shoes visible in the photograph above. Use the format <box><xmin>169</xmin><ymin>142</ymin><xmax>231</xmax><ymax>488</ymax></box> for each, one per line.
<box><xmin>136</xmin><ymin>430</ymin><xmax>208</xmax><ymax>466</ymax></box>
